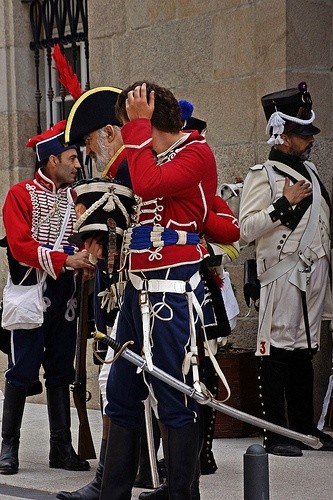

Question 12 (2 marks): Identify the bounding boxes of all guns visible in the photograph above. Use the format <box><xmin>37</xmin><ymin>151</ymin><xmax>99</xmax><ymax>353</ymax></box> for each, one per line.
<box><xmin>69</xmin><ymin>254</ymin><xmax>98</xmax><ymax>462</ymax></box>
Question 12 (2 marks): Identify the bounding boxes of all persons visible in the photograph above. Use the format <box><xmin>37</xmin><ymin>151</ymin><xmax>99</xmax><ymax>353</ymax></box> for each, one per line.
<box><xmin>57</xmin><ymin>81</ymin><xmax>216</xmax><ymax>500</ymax></box>
<box><xmin>0</xmin><ymin>121</ymin><xmax>95</xmax><ymax>474</ymax></box>
<box><xmin>65</xmin><ymin>88</ymin><xmax>153</xmax><ymax>491</ymax></box>
<box><xmin>238</xmin><ymin>84</ymin><xmax>333</xmax><ymax>456</ymax></box>
<box><xmin>158</xmin><ymin>101</ymin><xmax>243</xmax><ymax>477</ymax></box>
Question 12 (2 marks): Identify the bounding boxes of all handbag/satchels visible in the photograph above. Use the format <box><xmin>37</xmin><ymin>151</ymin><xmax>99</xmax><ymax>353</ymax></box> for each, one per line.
<box><xmin>1</xmin><ymin>284</ymin><xmax>50</xmax><ymax>331</ymax></box>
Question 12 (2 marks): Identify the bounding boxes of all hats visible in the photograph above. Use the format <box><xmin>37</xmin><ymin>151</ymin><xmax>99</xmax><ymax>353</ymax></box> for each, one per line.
<box><xmin>177</xmin><ymin>100</ymin><xmax>208</xmax><ymax>135</ymax></box>
<box><xmin>27</xmin><ymin>120</ymin><xmax>81</xmax><ymax>162</ymax></box>
<box><xmin>66</xmin><ymin>176</ymin><xmax>134</xmax><ymax>244</ymax></box>
<box><xmin>260</xmin><ymin>81</ymin><xmax>321</xmax><ymax>137</ymax></box>
<box><xmin>64</xmin><ymin>86</ymin><xmax>127</xmax><ymax>144</ymax></box>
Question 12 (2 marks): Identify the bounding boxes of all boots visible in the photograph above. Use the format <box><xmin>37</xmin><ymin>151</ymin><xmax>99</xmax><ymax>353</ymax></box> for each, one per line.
<box><xmin>283</xmin><ymin>350</ymin><xmax>333</xmax><ymax>451</ymax></box>
<box><xmin>159</xmin><ymin>363</ymin><xmax>218</xmax><ymax>475</ymax></box>
<box><xmin>99</xmin><ymin>417</ymin><xmax>138</xmax><ymax>500</ymax></box>
<box><xmin>138</xmin><ymin>416</ymin><xmax>199</xmax><ymax>500</ymax></box>
<box><xmin>0</xmin><ymin>381</ymin><xmax>26</xmax><ymax>474</ymax></box>
<box><xmin>47</xmin><ymin>387</ymin><xmax>91</xmax><ymax>471</ymax></box>
<box><xmin>154</xmin><ymin>421</ymin><xmax>203</xmax><ymax>500</ymax></box>
<box><xmin>57</xmin><ymin>437</ymin><xmax>106</xmax><ymax>500</ymax></box>
<box><xmin>134</xmin><ymin>411</ymin><xmax>163</xmax><ymax>488</ymax></box>
<box><xmin>255</xmin><ymin>345</ymin><xmax>304</xmax><ymax>457</ymax></box>
